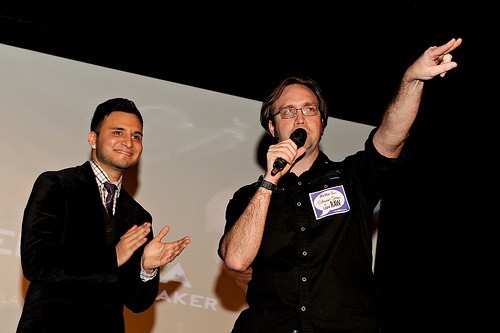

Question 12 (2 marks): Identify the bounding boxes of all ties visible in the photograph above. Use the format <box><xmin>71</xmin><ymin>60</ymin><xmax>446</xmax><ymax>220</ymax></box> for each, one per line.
<box><xmin>103</xmin><ymin>181</ymin><xmax>119</xmax><ymax>217</ymax></box>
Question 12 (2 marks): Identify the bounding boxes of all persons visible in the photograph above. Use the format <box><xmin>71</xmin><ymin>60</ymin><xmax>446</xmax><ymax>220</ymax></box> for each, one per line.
<box><xmin>218</xmin><ymin>38</ymin><xmax>462</xmax><ymax>333</ymax></box>
<box><xmin>15</xmin><ymin>98</ymin><xmax>190</xmax><ymax>333</ymax></box>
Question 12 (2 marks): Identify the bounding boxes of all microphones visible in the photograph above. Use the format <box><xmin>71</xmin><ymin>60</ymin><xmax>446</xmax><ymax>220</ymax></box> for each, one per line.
<box><xmin>271</xmin><ymin>128</ymin><xmax>307</xmax><ymax>176</ymax></box>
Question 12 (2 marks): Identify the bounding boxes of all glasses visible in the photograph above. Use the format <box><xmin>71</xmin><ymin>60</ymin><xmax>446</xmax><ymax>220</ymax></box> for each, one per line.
<box><xmin>276</xmin><ymin>104</ymin><xmax>320</xmax><ymax>120</ymax></box>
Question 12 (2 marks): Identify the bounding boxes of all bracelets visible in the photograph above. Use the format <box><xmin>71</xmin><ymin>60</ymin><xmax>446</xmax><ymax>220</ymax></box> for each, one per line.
<box><xmin>255</xmin><ymin>175</ymin><xmax>278</xmax><ymax>193</ymax></box>
